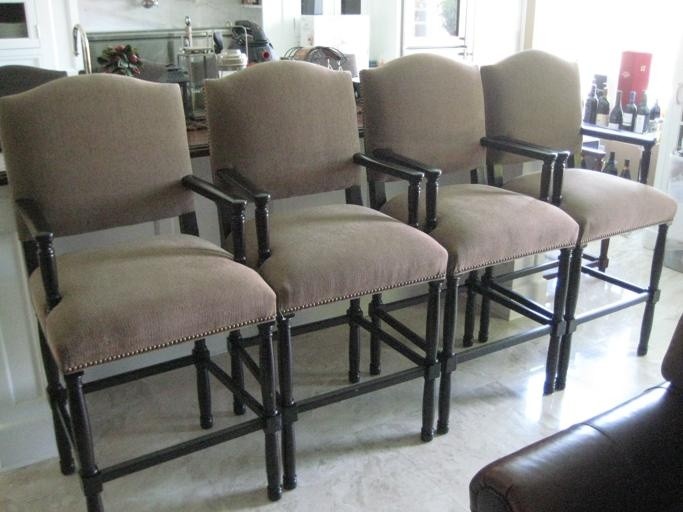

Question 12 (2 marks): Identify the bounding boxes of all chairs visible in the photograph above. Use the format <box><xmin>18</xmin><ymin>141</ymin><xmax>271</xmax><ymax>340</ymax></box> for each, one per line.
<box><xmin>359</xmin><ymin>52</ymin><xmax>579</xmax><ymax>437</ymax></box>
<box><xmin>1</xmin><ymin>72</ymin><xmax>283</xmax><ymax>512</ymax></box>
<box><xmin>201</xmin><ymin>56</ymin><xmax>451</xmax><ymax>491</ymax></box>
<box><xmin>477</xmin><ymin>49</ymin><xmax>679</xmax><ymax>391</ymax></box>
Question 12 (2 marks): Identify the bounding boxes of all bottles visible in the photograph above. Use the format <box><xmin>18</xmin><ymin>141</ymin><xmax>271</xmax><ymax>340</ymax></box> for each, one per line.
<box><xmin>583</xmin><ymin>83</ymin><xmax>663</xmax><ymax>136</ymax></box>
<box><xmin>601</xmin><ymin>151</ymin><xmax>632</xmax><ymax>180</ymax></box>
